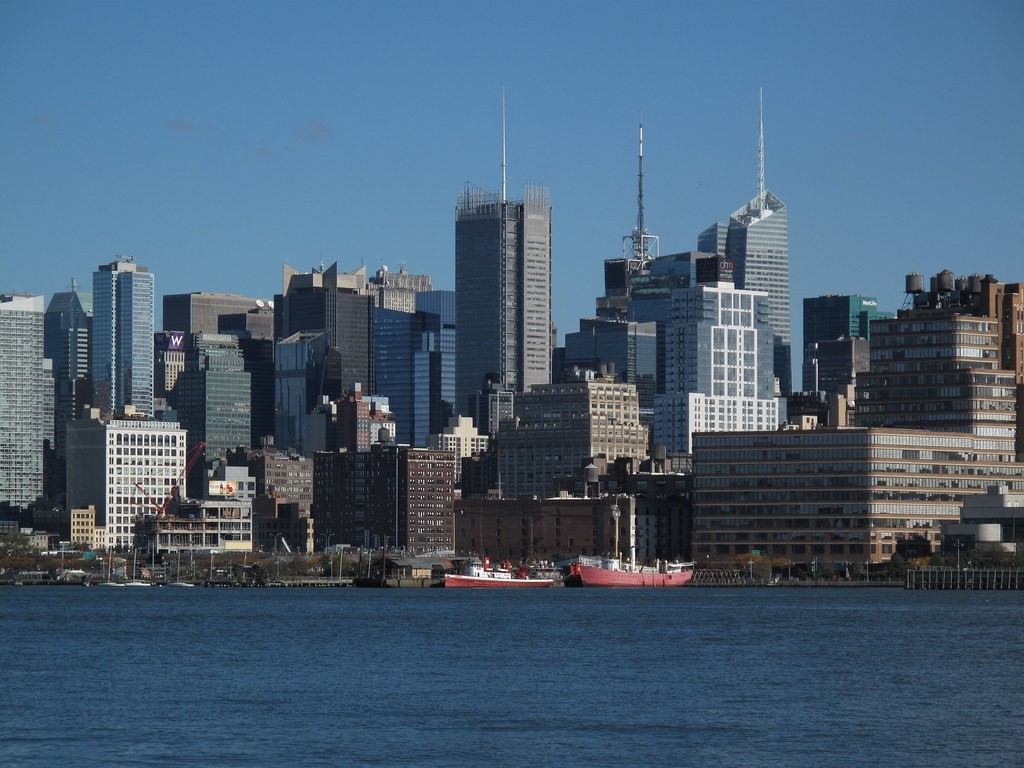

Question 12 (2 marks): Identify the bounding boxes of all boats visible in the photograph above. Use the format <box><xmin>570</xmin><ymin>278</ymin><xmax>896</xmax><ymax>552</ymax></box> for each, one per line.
<box><xmin>571</xmin><ymin>559</ymin><xmax>696</xmax><ymax>586</ymax></box>
<box><xmin>444</xmin><ymin>573</ymin><xmax>554</xmax><ymax>587</ymax></box>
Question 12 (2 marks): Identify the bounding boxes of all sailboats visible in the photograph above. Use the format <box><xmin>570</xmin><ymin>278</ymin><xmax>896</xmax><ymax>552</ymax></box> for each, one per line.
<box><xmin>98</xmin><ymin>546</ymin><xmax>125</xmax><ymax>587</ymax></box>
<box><xmin>125</xmin><ymin>548</ymin><xmax>150</xmax><ymax>587</ymax></box>
<box><xmin>167</xmin><ymin>552</ymin><xmax>194</xmax><ymax>586</ymax></box>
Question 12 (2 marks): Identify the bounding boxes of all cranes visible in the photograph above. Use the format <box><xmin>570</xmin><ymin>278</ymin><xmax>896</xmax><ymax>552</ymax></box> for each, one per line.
<box><xmin>133</xmin><ymin>442</ymin><xmax>208</xmax><ymax>520</ymax></box>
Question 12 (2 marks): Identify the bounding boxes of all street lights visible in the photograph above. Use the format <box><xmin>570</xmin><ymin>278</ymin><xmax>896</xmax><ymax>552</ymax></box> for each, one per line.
<box><xmin>865</xmin><ymin>559</ymin><xmax>870</xmax><ymax>581</ymax></box>
<box><xmin>269</xmin><ymin>533</ymin><xmax>282</xmax><ymax>552</ymax></box>
<box><xmin>144</xmin><ymin>534</ymin><xmax>149</xmax><ymax>553</ymax></box>
<box><xmin>328</xmin><ymin>534</ymin><xmax>334</xmax><ymax>547</ymax></box>
<box><xmin>220</xmin><ymin>533</ymin><xmax>230</xmax><ymax>553</ymax></box>
<box><xmin>59</xmin><ymin>541</ymin><xmax>70</xmax><ymax>570</ymax></box>
<box><xmin>321</xmin><ymin>534</ymin><xmax>327</xmax><ymax>552</ymax></box>
<box><xmin>368</xmin><ymin>549</ymin><xmax>375</xmax><ymax>578</ymax></box>
<box><xmin>611</xmin><ymin>505</ymin><xmax>621</xmax><ymax>558</ymax></box>
<box><xmin>748</xmin><ymin>559</ymin><xmax>754</xmax><ymax>578</ymax></box>
